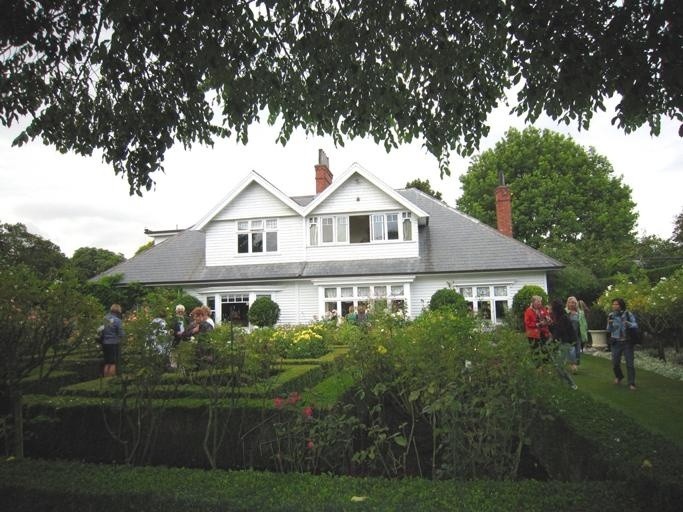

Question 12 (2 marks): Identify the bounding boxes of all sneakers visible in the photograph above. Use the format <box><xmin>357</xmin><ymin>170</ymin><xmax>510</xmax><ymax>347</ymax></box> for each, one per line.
<box><xmin>614</xmin><ymin>377</ymin><xmax>637</xmax><ymax>391</ymax></box>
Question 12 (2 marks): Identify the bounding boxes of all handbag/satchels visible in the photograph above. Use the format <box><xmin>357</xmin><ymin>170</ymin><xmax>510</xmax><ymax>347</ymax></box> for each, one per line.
<box><xmin>629</xmin><ymin>327</ymin><xmax>641</xmax><ymax>344</ymax></box>
<box><xmin>94</xmin><ymin>325</ymin><xmax>105</xmax><ymax>343</ymax></box>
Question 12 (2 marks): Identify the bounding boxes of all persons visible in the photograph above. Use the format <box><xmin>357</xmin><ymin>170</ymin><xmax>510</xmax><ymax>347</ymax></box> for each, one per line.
<box><xmin>522</xmin><ymin>295</ymin><xmax>589</xmax><ymax>391</ymax></box>
<box><xmin>101</xmin><ymin>303</ymin><xmax>125</xmax><ymax>377</ymax></box>
<box><xmin>168</xmin><ymin>305</ymin><xmax>214</xmax><ymax>361</ymax></box>
<box><xmin>605</xmin><ymin>298</ymin><xmax>639</xmax><ymax>390</ymax></box>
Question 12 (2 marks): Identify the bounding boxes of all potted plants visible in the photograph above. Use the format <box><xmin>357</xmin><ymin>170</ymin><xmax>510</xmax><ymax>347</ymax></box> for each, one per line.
<box><xmin>582</xmin><ymin>305</ymin><xmax>612</xmax><ymax>349</ymax></box>
